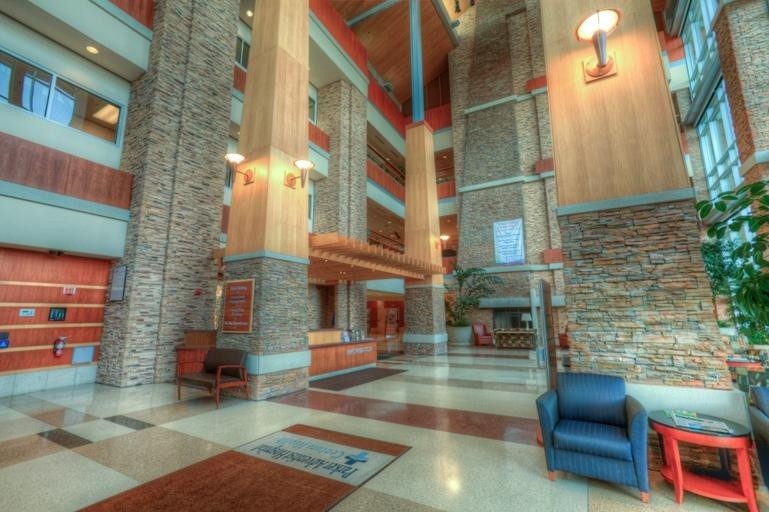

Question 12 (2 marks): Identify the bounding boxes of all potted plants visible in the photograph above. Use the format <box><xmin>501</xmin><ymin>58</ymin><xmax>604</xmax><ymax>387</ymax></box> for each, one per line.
<box><xmin>444</xmin><ymin>262</ymin><xmax>503</xmax><ymax>347</ymax></box>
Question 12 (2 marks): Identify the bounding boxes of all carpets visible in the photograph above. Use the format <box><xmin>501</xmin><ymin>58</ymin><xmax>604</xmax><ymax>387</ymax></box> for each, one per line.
<box><xmin>74</xmin><ymin>423</ymin><xmax>412</xmax><ymax>511</ymax></box>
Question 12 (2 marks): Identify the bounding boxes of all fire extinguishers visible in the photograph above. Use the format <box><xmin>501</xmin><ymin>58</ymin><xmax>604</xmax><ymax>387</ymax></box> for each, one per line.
<box><xmin>53</xmin><ymin>335</ymin><xmax>68</xmax><ymax>357</ymax></box>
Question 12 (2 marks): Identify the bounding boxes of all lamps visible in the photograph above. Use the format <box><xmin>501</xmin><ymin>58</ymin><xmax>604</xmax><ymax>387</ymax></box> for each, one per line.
<box><xmin>574</xmin><ymin>6</ymin><xmax>622</xmax><ymax>84</ymax></box>
<box><xmin>223</xmin><ymin>153</ymin><xmax>313</xmax><ymax>191</ymax></box>
<box><xmin>520</xmin><ymin>312</ymin><xmax>533</xmax><ymax>332</ymax></box>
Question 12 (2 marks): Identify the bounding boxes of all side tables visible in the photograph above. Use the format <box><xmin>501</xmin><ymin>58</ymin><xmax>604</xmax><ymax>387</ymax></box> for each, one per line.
<box><xmin>648</xmin><ymin>409</ymin><xmax>761</xmax><ymax>511</ymax></box>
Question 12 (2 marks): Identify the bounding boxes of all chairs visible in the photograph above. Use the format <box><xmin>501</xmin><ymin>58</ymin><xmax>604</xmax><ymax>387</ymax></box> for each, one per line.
<box><xmin>472</xmin><ymin>321</ymin><xmax>494</xmax><ymax>347</ymax></box>
<box><xmin>535</xmin><ymin>372</ymin><xmax>650</xmax><ymax>504</ymax></box>
<box><xmin>176</xmin><ymin>348</ymin><xmax>251</xmax><ymax>409</ymax></box>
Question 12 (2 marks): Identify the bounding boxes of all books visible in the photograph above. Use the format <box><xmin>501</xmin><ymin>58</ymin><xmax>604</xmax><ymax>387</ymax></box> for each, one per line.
<box><xmin>663</xmin><ymin>405</ymin><xmax>736</xmax><ymax>435</ymax></box>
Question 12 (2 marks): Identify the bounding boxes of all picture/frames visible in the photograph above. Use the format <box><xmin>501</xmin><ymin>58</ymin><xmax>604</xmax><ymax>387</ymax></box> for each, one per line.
<box><xmin>48</xmin><ymin>307</ymin><xmax>67</xmax><ymax>321</ymax></box>
<box><xmin>221</xmin><ymin>278</ymin><xmax>255</xmax><ymax>334</ymax></box>
